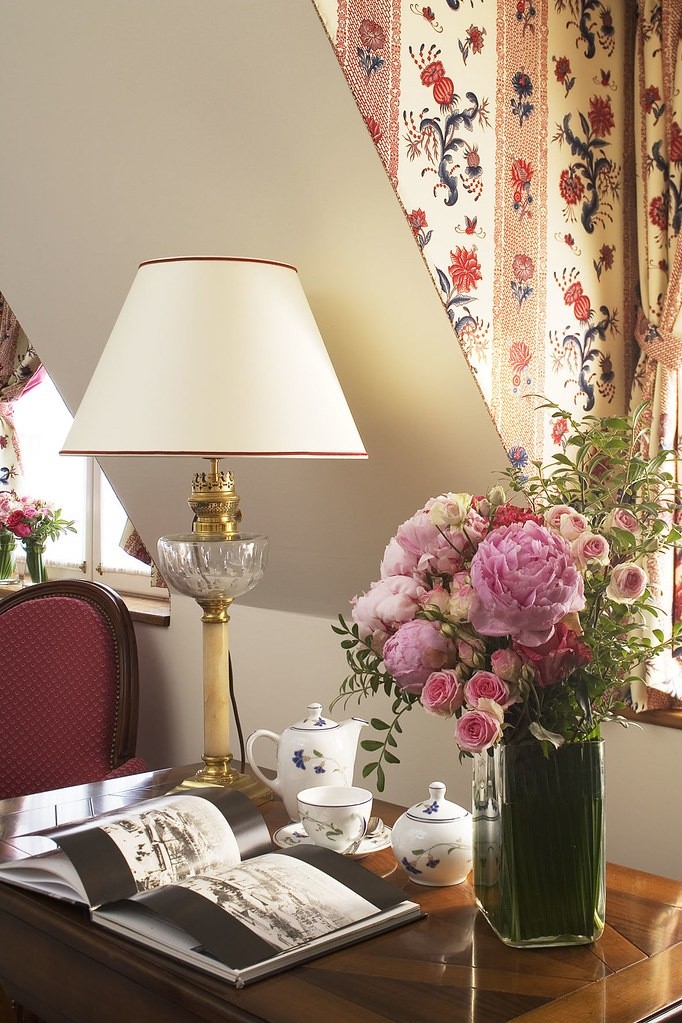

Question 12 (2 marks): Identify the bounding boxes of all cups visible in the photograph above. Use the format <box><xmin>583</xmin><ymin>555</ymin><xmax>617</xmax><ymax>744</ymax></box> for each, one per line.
<box><xmin>390</xmin><ymin>781</ymin><xmax>472</xmax><ymax>886</ymax></box>
<box><xmin>296</xmin><ymin>785</ymin><xmax>373</xmax><ymax>851</ymax></box>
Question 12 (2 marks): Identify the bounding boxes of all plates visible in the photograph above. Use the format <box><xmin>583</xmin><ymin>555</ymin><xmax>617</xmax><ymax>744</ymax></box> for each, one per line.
<box><xmin>272</xmin><ymin>822</ymin><xmax>393</xmax><ymax>859</ymax></box>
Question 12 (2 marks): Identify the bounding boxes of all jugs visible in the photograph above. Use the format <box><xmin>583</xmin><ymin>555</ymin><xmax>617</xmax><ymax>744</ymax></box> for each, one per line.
<box><xmin>245</xmin><ymin>703</ymin><xmax>369</xmax><ymax>822</ymax></box>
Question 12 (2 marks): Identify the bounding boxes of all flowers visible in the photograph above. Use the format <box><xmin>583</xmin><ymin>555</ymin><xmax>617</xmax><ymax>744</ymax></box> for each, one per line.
<box><xmin>0</xmin><ymin>467</ymin><xmax>27</xmax><ymax>534</ymax></box>
<box><xmin>327</xmin><ymin>397</ymin><xmax>682</xmax><ymax>794</ymax></box>
<box><xmin>9</xmin><ymin>508</ymin><xmax>77</xmax><ymax>582</ymax></box>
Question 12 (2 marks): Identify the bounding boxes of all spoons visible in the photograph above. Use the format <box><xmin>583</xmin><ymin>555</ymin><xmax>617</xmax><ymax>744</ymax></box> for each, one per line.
<box><xmin>344</xmin><ymin>817</ymin><xmax>384</xmax><ymax>855</ymax></box>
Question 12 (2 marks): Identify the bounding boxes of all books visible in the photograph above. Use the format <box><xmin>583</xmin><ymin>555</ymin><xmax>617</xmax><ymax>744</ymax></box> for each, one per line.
<box><xmin>0</xmin><ymin>787</ymin><xmax>428</xmax><ymax>987</ymax></box>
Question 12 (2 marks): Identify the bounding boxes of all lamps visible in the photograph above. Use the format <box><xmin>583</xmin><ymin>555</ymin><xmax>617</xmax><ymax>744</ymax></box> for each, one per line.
<box><xmin>60</xmin><ymin>256</ymin><xmax>374</xmax><ymax>830</ymax></box>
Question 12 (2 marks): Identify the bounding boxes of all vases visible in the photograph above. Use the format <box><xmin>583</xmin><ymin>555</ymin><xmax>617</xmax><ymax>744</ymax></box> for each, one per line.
<box><xmin>21</xmin><ymin>547</ymin><xmax>51</xmax><ymax>588</ymax></box>
<box><xmin>0</xmin><ymin>532</ymin><xmax>20</xmax><ymax>585</ymax></box>
<box><xmin>468</xmin><ymin>745</ymin><xmax>606</xmax><ymax>950</ymax></box>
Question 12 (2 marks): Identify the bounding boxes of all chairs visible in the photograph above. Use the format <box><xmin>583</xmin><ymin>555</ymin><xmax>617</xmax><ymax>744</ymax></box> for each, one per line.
<box><xmin>0</xmin><ymin>578</ymin><xmax>150</xmax><ymax>800</ymax></box>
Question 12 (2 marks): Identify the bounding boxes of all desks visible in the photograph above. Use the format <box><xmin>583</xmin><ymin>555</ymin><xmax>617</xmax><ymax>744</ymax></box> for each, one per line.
<box><xmin>1</xmin><ymin>761</ymin><xmax>682</xmax><ymax>1023</ymax></box>
